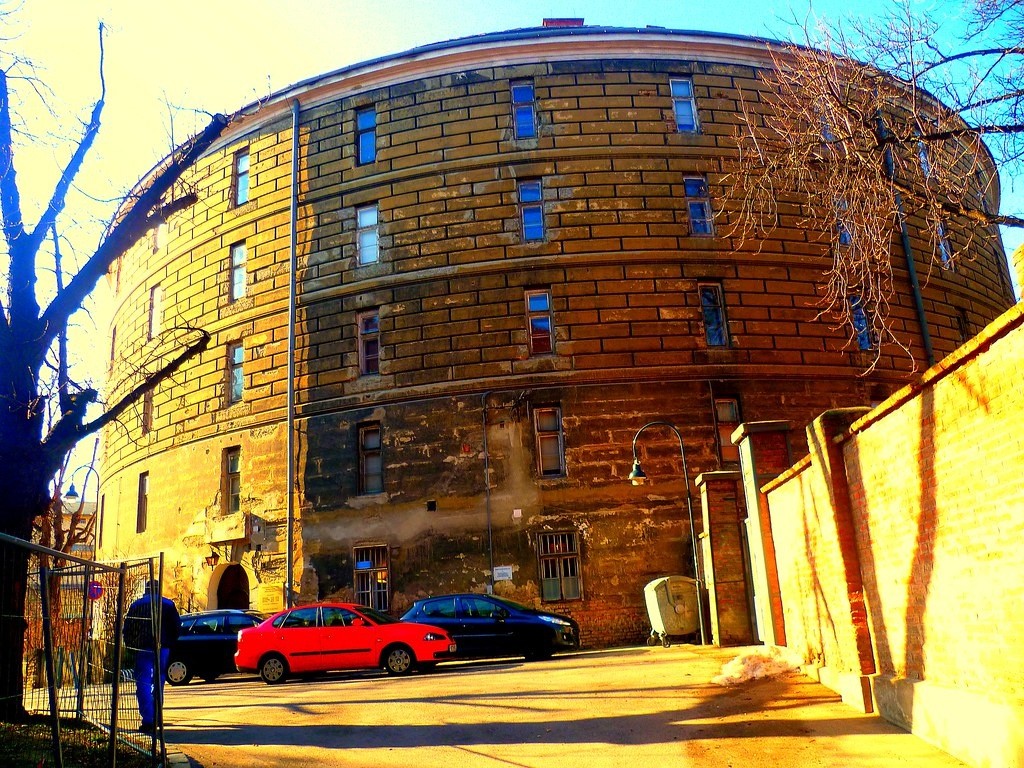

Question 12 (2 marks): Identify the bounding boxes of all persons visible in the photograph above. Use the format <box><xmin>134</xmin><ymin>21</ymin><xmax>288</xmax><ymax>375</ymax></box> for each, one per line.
<box><xmin>122</xmin><ymin>579</ymin><xmax>182</xmax><ymax>730</ymax></box>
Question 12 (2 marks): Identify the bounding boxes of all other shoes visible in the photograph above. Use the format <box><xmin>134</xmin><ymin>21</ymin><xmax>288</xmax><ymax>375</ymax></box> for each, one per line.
<box><xmin>139</xmin><ymin>725</ymin><xmax>157</xmax><ymax>735</ymax></box>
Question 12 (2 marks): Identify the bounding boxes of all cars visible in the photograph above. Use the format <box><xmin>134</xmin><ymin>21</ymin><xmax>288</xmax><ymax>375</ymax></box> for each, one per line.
<box><xmin>401</xmin><ymin>594</ymin><xmax>580</xmax><ymax>660</ymax></box>
<box><xmin>234</xmin><ymin>604</ymin><xmax>457</xmax><ymax>684</ymax></box>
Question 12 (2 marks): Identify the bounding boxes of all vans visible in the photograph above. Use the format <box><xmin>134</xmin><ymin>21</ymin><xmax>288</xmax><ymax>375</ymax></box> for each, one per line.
<box><xmin>166</xmin><ymin>610</ymin><xmax>268</xmax><ymax>687</ymax></box>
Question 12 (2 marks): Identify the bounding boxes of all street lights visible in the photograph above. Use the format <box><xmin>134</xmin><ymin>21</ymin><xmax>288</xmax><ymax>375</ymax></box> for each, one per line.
<box><xmin>628</xmin><ymin>421</ymin><xmax>710</xmax><ymax>646</ymax></box>
<box><xmin>63</xmin><ymin>465</ymin><xmax>99</xmax><ymax>627</ymax></box>
